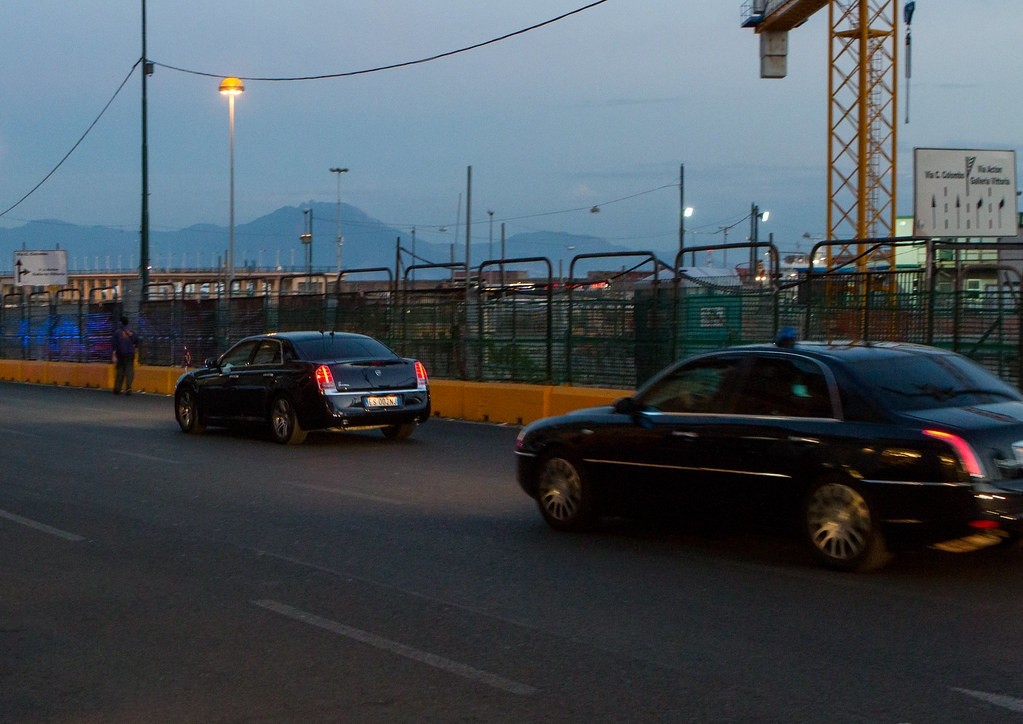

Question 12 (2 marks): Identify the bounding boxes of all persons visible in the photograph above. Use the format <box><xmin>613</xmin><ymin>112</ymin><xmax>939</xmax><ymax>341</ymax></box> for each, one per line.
<box><xmin>111</xmin><ymin>316</ymin><xmax>138</xmax><ymax>396</ymax></box>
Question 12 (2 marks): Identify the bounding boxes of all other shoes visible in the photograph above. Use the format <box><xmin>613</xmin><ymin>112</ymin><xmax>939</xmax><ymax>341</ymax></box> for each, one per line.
<box><xmin>125</xmin><ymin>387</ymin><xmax>132</xmax><ymax>395</ymax></box>
<box><xmin>113</xmin><ymin>388</ymin><xmax>120</xmax><ymax>394</ymax></box>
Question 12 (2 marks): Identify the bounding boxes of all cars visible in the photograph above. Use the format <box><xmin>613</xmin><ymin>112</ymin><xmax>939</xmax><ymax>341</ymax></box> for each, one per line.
<box><xmin>513</xmin><ymin>340</ymin><xmax>1023</xmax><ymax>574</ymax></box>
<box><xmin>173</xmin><ymin>330</ymin><xmax>432</xmax><ymax>444</ymax></box>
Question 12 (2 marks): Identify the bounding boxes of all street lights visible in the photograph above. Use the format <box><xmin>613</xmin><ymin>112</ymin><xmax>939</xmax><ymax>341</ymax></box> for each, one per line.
<box><xmin>750</xmin><ymin>212</ymin><xmax>770</xmax><ymax>275</ymax></box>
<box><xmin>219</xmin><ymin>77</ymin><xmax>244</xmax><ymax>299</ymax></box>
<box><xmin>679</xmin><ymin>207</ymin><xmax>694</xmax><ymax>267</ymax></box>
<box><xmin>330</xmin><ymin>167</ymin><xmax>349</xmax><ymax>271</ymax></box>
